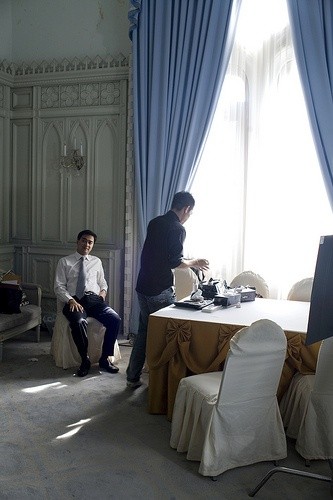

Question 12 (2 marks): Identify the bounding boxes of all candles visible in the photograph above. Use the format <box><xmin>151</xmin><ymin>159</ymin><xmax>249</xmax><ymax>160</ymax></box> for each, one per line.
<box><xmin>63</xmin><ymin>139</ymin><xmax>83</xmax><ymax>157</ymax></box>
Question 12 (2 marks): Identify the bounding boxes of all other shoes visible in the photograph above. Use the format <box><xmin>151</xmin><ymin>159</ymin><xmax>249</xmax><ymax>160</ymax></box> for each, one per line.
<box><xmin>127</xmin><ymin>380</ymin><xmax>143</xmax><ymax>387</ymax></box>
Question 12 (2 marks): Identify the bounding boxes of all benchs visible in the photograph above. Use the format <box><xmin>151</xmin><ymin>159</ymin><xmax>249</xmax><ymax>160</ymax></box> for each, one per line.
<box><xmin>0</xmin><ymin>283</ymin><xmax>42</xmax><ymax>343</ymax></box>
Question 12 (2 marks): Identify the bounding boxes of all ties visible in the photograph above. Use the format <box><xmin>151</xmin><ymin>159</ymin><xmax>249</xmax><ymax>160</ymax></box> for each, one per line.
<box><xmin>75</xmin><ymin>256</ymin><xmax>85</xmax><ymax>300</ymax></box>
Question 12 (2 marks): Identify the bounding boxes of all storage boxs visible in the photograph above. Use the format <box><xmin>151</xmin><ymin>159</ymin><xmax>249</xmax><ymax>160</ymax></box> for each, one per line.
<box><xmin>0</xmin><ymin>269</ymin><xmax>30</xmax><ymax>315</ymax></box>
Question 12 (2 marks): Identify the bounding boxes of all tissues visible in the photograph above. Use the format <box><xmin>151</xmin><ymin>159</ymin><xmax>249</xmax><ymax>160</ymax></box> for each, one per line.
<box><xmin>214</xmin><ymin>281</ymin><xmax>241</xmax><ymax>307</ymax></box>
<box><xmin>233</xmin><ymin>284</ymin><xmax>256</xmax><ymax>302</ymax></box>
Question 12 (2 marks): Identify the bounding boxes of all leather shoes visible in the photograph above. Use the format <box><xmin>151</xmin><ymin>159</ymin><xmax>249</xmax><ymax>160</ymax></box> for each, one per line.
<box><xmin>99</xmin><ymin>359</ymin><xmax>119</xmax><ymax>372</ymax></box>
<box><xmin>77</xmin><ymin>358</ymin><xmax>90</xmax><ymax>377</ymax></box>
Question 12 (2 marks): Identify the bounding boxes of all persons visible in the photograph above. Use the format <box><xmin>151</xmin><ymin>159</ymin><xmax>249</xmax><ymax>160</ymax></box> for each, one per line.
<box><xmin>125</xmin><ymin>191</ymin><xmax>210</xmax><ymax>389</ymax></box>
<box><xmin>54</xmin><ymin>230</ymin><xmax>121</xmax><ymax>377</ymax></box>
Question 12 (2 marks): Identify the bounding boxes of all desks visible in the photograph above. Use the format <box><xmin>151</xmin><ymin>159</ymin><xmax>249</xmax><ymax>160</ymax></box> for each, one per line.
<box><xmin>146</xmin><ymin>294</ymin><xmax>323</xmax><ymax>423</ymax></box>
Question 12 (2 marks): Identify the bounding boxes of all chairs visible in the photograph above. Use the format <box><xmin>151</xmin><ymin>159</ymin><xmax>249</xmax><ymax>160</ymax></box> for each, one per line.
<box><xmin>50</xmin><ymin>297</ymin><xmax>122</xmax><ymax>370</ymax></box>
<box><xmin>174</xmin><ymin>267</ymin><xmax>333</xmax><ymax>482</ymax></box>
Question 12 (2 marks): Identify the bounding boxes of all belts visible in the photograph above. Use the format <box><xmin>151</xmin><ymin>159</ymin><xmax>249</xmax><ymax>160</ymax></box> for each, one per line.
<box><xmin>84</xmin><ymin>291</ymin><xmax>95</xmax><ymax>295</ymax></box>
